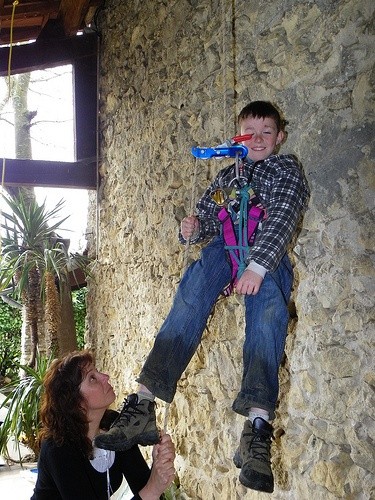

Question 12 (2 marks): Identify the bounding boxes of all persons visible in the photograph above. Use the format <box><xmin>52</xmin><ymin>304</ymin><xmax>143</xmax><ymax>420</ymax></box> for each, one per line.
<box><xmin>29</xmin><ymin>351</ymin><xmax>178</xmax><ymax>500</ymax></box>
<box><xmin>91</xmin><ymin>101</ymin><xmax>314</xmax><ymax>493</ymax></box>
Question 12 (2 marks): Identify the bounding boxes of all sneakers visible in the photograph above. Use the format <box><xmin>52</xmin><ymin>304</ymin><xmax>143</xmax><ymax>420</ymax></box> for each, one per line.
<box><xmin>93</xmin><ymin>393</ymin><xmax>160</xmax><ymax>451</ymax></box>
<box><xmin>233</xmin><ymin>417</ymin><xmax>274</xmax><ymax>493</ymax></box>
